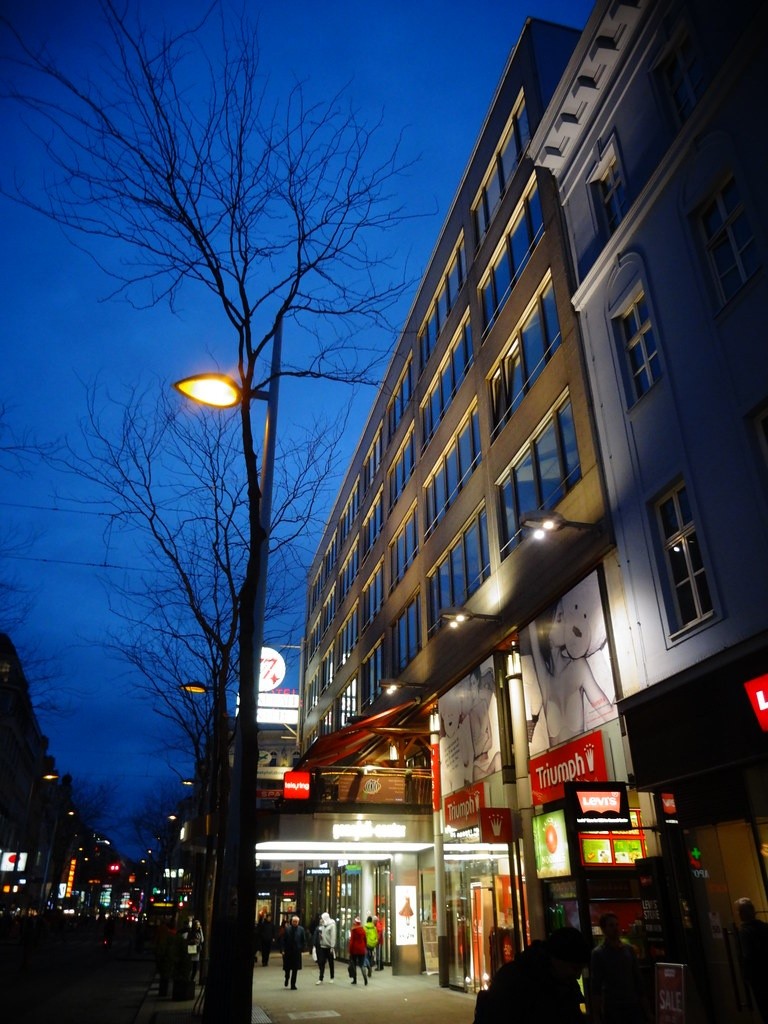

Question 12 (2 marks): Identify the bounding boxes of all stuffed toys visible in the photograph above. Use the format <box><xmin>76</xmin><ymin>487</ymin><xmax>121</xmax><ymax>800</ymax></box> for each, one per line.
<box><xmin>438</xmin><ymin>698</ymin><xmax>470</xmax><ymax>796</ymax></box>
<box><xmin>527</xmin><ymin>570</ymin><xmax>619</xmax><ymax>756</ymax></box>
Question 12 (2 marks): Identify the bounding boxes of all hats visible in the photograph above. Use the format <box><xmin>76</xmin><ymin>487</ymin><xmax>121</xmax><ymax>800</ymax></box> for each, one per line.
<box><xmin>352</xmin><ymin>916</ymin><xmax>361</xmax><ymax>925</ymax></box>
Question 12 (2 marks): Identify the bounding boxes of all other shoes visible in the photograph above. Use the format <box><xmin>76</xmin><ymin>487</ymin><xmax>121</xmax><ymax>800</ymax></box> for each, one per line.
<box><xmin>315</xmin><ymin>980</ymin><xmax>322</xmax><ymax>985</ymax></box>
<box><xmin>262</xmin><ymin>962</ymin><xmax>268</xmax><ymax>967</ymax></box>
<box><xmin>328</xmin><ymin>979</ymin><xmax>334</xmax><ymax>983</ymax></box>
<box><xmin>349</xmin><ymin>978</ymin><xmax>357</xmax><ymax>984</ymax></box>
<box><xmin>284</xmin><ymin>979</ymin><xmax>289</xmax><ymax>986</ymax></box>
<box><xmin>363</xmin><ymin>979</ymin><xmax>369</xmax><ymax>985</ymax></box>
<box><xmin>290</xmin><ymin>986</ymin><xmax>297</xmax><ymax>989</ymax></box>
<box><xmin>375</xmin><ymin>966</ymin><xmax>384</xmax><ymax>971</ymax></box>
<box><xmin>368</xmin><ymin>967</ymin><xmax>372</xmax><ymax>977</ymax></box>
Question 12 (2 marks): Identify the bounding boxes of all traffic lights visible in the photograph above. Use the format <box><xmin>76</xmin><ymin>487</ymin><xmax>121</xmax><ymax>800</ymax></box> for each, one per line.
<box><xmin>108</xmin><ymin>863</ymin><xmax>122</xmax><ymax>872</ymax></box>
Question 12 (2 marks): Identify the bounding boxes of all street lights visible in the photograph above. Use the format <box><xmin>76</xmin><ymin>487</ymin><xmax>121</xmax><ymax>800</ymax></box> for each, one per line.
<box><xmin>37</xmin><ymin>808</ymin><xmax>76</xmax><ymax>907</ymax></box>
<box><xmin>172</xmin><ymin>316</ymin><xmax>287</xmax><ymax>1023</ymax></box>
<box><xmin>10</xmin><ymin>768</ymin><xmax>62</xmax><ymax>896</ymax></box>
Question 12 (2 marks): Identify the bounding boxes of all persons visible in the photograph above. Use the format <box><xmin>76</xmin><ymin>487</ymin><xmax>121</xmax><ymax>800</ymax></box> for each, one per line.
<box><xmin>527</xmin><ymin>598</ymin><xmax>621</xmax><ymax>747</ymax></box>
<box><xmin>471</xmin><ymin>926</ymin><xmax>594</xmax><ymax>1023</ymax></box>
<box><xmin>374</xmin><ymin>915</ymin><xmax>384</xmax><ymax>970</ymax></box>
<box><xmin>186</xmin><ymin>919</ymin><xmax>204</xmax><ymax>982</ymax></box>
<box><xmin>255</xmin><ymin>913</ymin><xmax>277</xmax><ymax>966</ymax></box>
<box><xmin>255</xmin><ymin>912</ymin><xmax>322</xmax><ymax>955</ymax></box>
<box><xmin>363</xmin><ymin>915</ymin><xmax>379</xmax><ymax>976</ymax></box>
<box><xmin>463</xmin><ymin>666</ymin><xmax>502</xmax><ymax>784</ymax></box>
<box><xmin>591</xmin><ymin>911</ymin><xmax>653</xmax><ymax>1023</ymax></box>
<box><xmin>734</xmin><ymin>896</ymin><xmax>768</xmax><ymax>1024</ymax></box>
<box><xmin>313</xmin><ymin>911</ymin><xmax>338</xmax><ymax>985</ymax></box>
<box><xmin>348</xmin><ymin>916</ymin><xmax>367</xmax><ymax>984</ymax></box>
<box><xmin>279</xmin><ymin>915</ymin><xmax>305</xmax><ymax>989</ymax></box>
<box><xmin>178</xmin><ymin>916</ymin><xmax>192</xmax><ymax>939</ymax></box>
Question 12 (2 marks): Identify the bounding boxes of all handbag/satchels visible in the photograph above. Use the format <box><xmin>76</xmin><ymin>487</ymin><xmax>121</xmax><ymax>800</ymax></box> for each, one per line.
<box><xmin>187</xmin><ymin>944</ymin><xmax>198</xmax><ymax>955</ymax></box>
<box><xmin>348</xmin><ymin>954</ymin><xmax>355</xmax><ymax>977</ymax></box>
